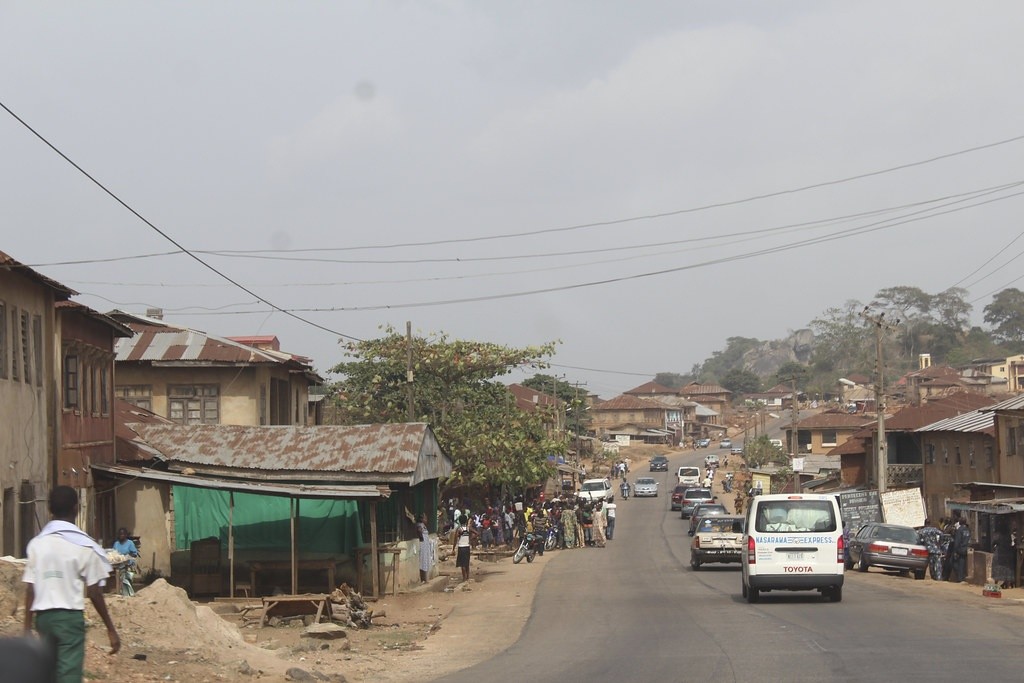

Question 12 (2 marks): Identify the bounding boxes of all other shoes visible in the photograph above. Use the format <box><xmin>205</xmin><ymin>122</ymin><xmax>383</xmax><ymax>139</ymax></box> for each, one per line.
<box><xmin>606</xmin><ymin>537</ymin><xmax>613</xmax><ymax>540</ymax></box>
<box><xmin>422</xmin><ymin>581</ymin><xmax>428</xmax><ymax>584</ymax></box>
<box><xmin>598</xmin><ymin>545</ymin><xmax>605</xmax><ymax>548</ymax></box>
<box><xmin>591</xmin><ymin>543</ymin><xmax>594</xmax><ymax>546</ymax></box>
<box><xmin>556</xmin><ymin>545</ymin><xmax>560</xmax><ymax>548</ymax></box>
<box><xmin>560</xmin><ymin>547</ymin><xmax>562</xmax><ymax>549</ymax></box>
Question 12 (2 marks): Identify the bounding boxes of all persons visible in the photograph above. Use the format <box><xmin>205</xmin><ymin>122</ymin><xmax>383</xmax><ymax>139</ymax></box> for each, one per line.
<box><xmin>441</xmin><ymin>489</ymin><xmax>616</xmax><ymax>550</ymax></box>
<box><xmin>20</xmin><ymin>485</ymin><xmax>120</xmax><ymax>683</ymax></box>
<box><xmin>915</xmin><ymin>517</ymin><xmax>973</xmax><ymax>583</ymax></box>
<box><xmin>618</xmin><ymin>479</ymin><xmax>631</xmax><ymax>496</ymax></box>
<box><xmin>452</xmin><ymin>514</ymin><xmax>480</xmax><ymax>582</ymax></box>
<box><xmin>415</xmin><ymin>513</ymin><xmax>431</xmax><ymax>584</ymax></box>
<box><xmin>0</xmin><ymin>632</ymin><xmax>56</xmax><ymax>683</ymax></box>
<box><xmin>734</xmin><ymin>492</ymin><xmax>743</xmax><ymax>515</ymax></box>
<box><xmin>702</xmin><ymin>455</ymin><xmax>734</xmax><ymax>490</ymax></box>
<box><xmin>991</xmin><ymin>518</ymin><xmax>1015</xmax><ymax>589</ymax></box>
<box><xmin>610</xmin><ymin>455</ymin><xmax>632</xmax><ymax>478</ymax></box>
<box><xmin>113</xmin><ymin>528</ymin><xmax>138</xmax><ymax>596</ymax></box>
<box><xmin>577</xmin><ymin>463</ymin><xmax>586</xmax><ymax>484</ymax></box>
<box><xmin>744</xmin><ymin>480</ymin><xmax>754</xmax><ymax>497</ymax></box>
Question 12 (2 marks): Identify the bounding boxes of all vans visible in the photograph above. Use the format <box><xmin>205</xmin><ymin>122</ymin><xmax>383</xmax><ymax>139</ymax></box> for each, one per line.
<box><xmin>740</xmin><ymin>494</ymin><xmax>844</xmax><ymax>601</ymax></box>
<box><xmin>675</xmin><ymin>466</ymin><xmax>701</xmax><ymax>485</ymax></box>
<box><xmin>769</xmin><ymin>439</ymin><xmax>782</xmax><ymax>451</ymax></box>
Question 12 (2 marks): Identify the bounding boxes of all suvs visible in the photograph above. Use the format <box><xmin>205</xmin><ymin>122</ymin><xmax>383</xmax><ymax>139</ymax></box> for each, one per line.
<box><xmin>578</xmin><ymin>478</ymin><xmax>615</xmax><ymax>505</ymax></box>
<box><xmin>689</xmin><ymin>503</ymin><xmax>731</xmax><ymax>537</ymax></box>
<box><xmin>681</xmin><ymin>487</ymin><xmax>718</xmax><ymax>520</ymax></box>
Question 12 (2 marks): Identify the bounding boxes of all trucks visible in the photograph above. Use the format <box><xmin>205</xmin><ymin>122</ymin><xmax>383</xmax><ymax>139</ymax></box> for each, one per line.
<box><xmin>704</xmin><ymin>454</ymin><xmax>720</xmax><ymax>468</ymax></box>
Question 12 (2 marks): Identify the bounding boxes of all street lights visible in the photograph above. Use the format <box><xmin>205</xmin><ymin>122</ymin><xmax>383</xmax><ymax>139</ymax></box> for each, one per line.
<box><xmin>577</xmin><ymin>405</ymin><xmax>591</xmax><ymax>467</ymax></box>
<box><xmin>836</xmin><ymin>375</ymin><xmax>887</xmax><ymax>502</ymax></box>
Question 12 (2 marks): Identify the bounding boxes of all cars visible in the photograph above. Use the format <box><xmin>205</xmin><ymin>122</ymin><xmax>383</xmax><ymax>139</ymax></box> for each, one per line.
<box><xmin>720</xmin><ymin>438</ymin><xmax>732</xmax><ymax>448</ymax></box>
<box><xmin>669</xmin><ymin>484</ymin><xmax>702</xmax><ymax>511</ymax></box>
<box><xmin>648</xmin><ymin>456</ymin><xmax>669</xmax><ymax>472</ymax></box>
<box><xmin>731</xmin><ymin>446</ymin><xmax>743</xmax><ymax>455</ymax></box>
<box><xmin>697</xmin><ymin>439</ymin><xmax>709</xmax><ymax>448</ymax></box>
<box><xmin>632</xmin><ymin>477</ymin><xmax>660</xmax><ymax>497</ymax></box>
<box><xmin>691</xmin><ymin>514</ymin><xmax>746</xmax><ymax>571</ymax></box>
<box><xmin>845</xmin><ymin>522</ymin><xmax>930</xmax><ymax>580</ymax></box>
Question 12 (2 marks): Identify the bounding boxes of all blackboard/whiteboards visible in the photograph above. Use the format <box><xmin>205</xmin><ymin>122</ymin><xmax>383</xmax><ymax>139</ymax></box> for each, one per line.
<box><xmin>839</xmin><ymin>489</ymin><xmax>882</xmax><ymax>539</ymax></box>
<box><xmin>879</xmin><ymin>487</ymin><xmax>926</xmax><ymax>527</ymax></box>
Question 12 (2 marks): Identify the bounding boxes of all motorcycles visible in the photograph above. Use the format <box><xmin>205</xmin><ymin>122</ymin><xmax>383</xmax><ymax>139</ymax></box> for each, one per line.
<box><xmin>513</xmin><ymin>532</ymin><xmax>543</xmax><ymax>565</ymax></box>
<box><xmin>543</xmin><ymin>528</ymin><xmax>558</xmax><ymax>551</ymax></box>
